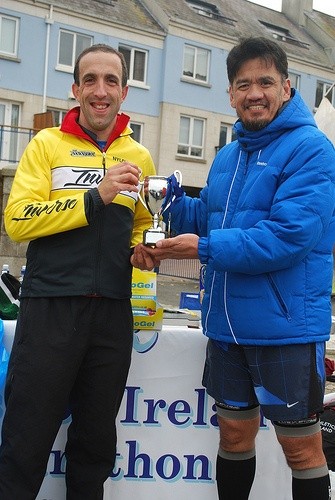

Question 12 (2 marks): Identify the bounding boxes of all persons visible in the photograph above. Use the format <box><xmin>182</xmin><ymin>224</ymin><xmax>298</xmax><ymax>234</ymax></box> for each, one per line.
<box><xmin>0</xmin><ymin>43</ymin><xmax>161</xmax><ymax>500</ymax></box>
<box><xmin>141</xmin><ymin>39</ymin><xmax>335</xmax><ymax>500</ymax></box>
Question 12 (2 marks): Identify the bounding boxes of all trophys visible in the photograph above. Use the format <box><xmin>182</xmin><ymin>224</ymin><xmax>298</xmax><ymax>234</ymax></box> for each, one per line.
<box><xmin>132</xmin><ymin>163</ymin><xmax>183</xmax><ymax>245</ymax></box>
